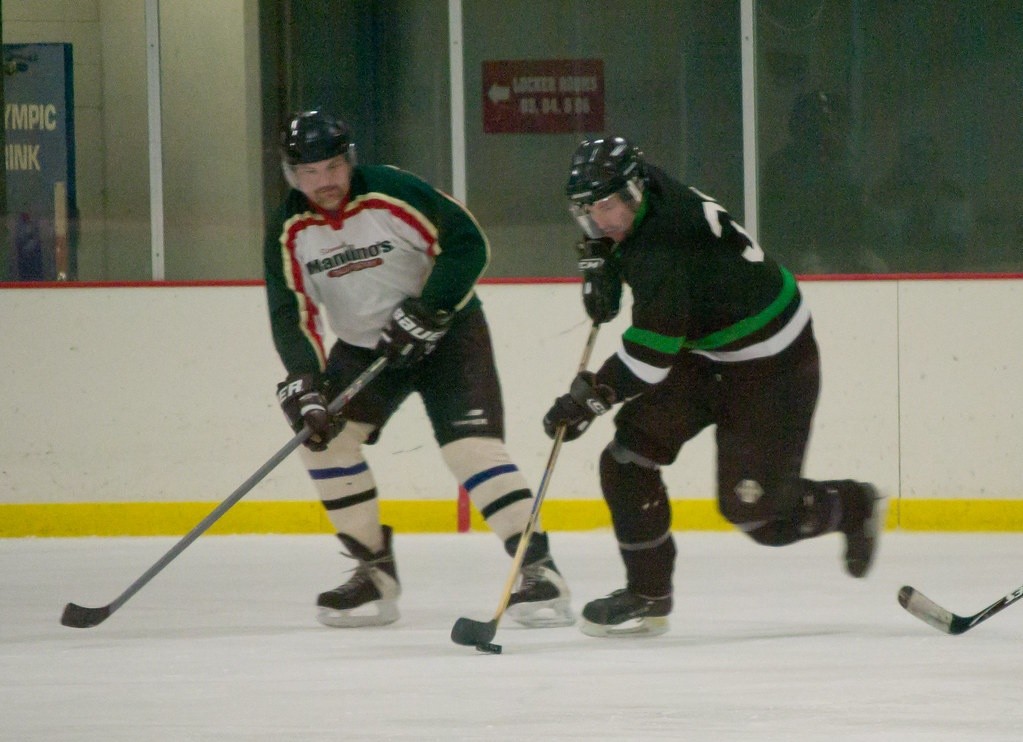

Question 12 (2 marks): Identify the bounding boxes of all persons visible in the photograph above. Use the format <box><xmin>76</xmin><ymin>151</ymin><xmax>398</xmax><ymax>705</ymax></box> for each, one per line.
<box><xmin>544</xmin><ymin>136</ymin><xmax>880</xmax><ymax>640</ymax></box>
<box><xmin>261</xmin><ymin>109</ymin><xmax>577</xmax><ymax>628</ymax></box>
<box><xmin>759</xmin><ymin>90</ymin><xmax>889</xmax><ymax>274</ymax></box>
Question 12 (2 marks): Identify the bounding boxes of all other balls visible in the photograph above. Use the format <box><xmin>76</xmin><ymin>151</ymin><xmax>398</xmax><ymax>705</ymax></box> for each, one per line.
<box><xmin>472</xmin><ymin>641</ymin><xmax>502</xmax><ymax>653</ymax></box>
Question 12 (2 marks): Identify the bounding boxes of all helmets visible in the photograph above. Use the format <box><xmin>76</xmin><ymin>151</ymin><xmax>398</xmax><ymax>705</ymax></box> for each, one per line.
<box><xmin>563</xmin><ymin>135</ymin><xmax>646</xmax><ymax>212</ymax></box>
<box><xmin>282</xmin><ymin>109</ymin><xmax>351</xmax><ymax>164</ymax></box>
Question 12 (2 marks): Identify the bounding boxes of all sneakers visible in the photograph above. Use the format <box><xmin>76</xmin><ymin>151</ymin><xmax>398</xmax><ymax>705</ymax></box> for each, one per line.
<box><xmin>313</xmin><ymin>523</ymin><xmax>401</xmax><ymax>626</ymax></box>
<box><xmin>577</xmin><ymin>582</ymin><xmax>677</xmax><ymax>636</ymax></box>
<box><xmin>831</xmin><ymin>474</ymin><xmax>880</xmax><ymax>576</ymax></box>
<box><xmin>500</xmin><ymin>530</ymin><xmax>576</xmax><ymax>629</ymax></box>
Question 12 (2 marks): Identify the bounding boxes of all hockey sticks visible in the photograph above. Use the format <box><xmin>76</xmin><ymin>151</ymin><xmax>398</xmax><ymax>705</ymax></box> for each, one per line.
<box><xmin>58</xmin><ymin>356</ymin><xmax>386</xmax><ymax>629</ymax></box>
<box><xmin>448</xmin><ymin>315</ymin><xmax>601</xmax><ymax>648</ymax></box>
<box><xmin>899</xmin><ymin>583</ymin><xmax>1023</xmax><ymax>637</ymax></box>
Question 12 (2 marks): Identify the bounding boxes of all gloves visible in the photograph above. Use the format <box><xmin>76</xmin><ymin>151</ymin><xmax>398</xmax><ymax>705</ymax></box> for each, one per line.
<box><xmin>276</xmin><ymin>369</ymin><xmax>348</xmax><ymax>452</ymax></box>
<box><xmin>575</xmin><ymin>235</ymin><xmax>621</xmax><ymax>322</ymax></box>
<box><xmin>543</xmin><ymin>368</ymin><xmax>611</xmax><ymax>442</ymax></box>
<box><xmin>373</xmin><ymin>293</ymin><xmax>460</xmax><ymax>367</ymax></box>
